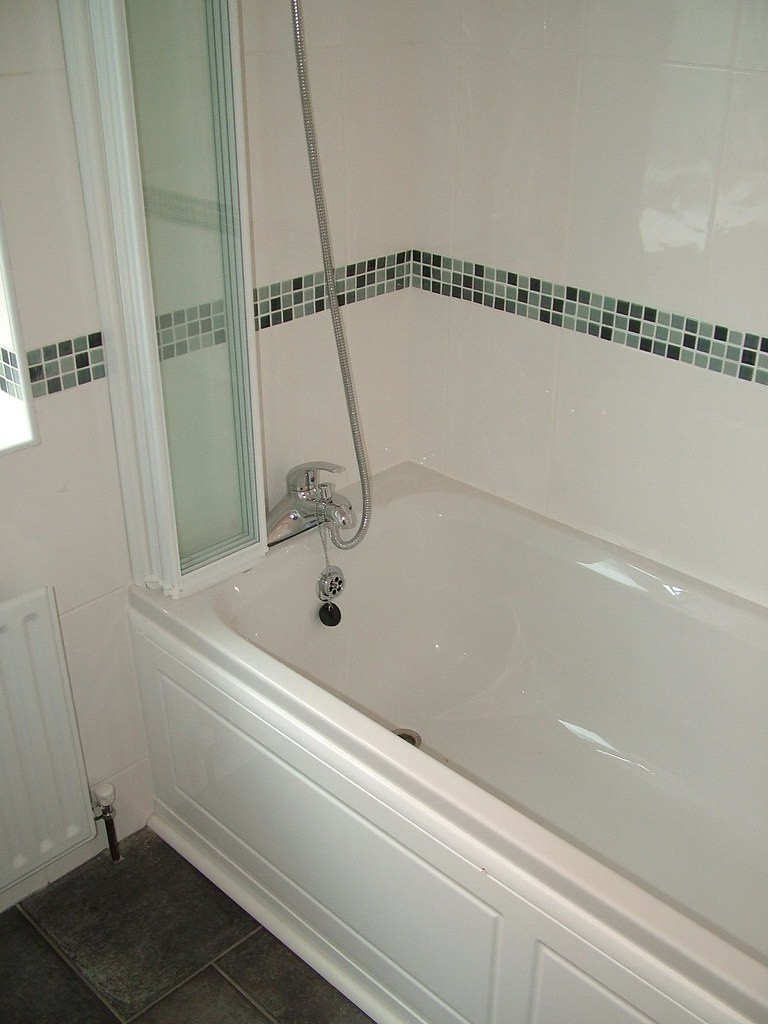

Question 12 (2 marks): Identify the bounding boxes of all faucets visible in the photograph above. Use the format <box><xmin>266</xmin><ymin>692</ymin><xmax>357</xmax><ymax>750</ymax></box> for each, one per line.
<box><xmin>265</xmin><ymin>460</ymin><xmax>358</xmax><ymax>548</ymax></box>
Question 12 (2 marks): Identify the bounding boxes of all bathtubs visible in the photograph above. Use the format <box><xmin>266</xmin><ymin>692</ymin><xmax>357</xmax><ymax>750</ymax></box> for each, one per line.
<box><xmin>125</xmin><ymin>458</ymin><xmax>767</xmax><ymax>1023</ymax></box>
